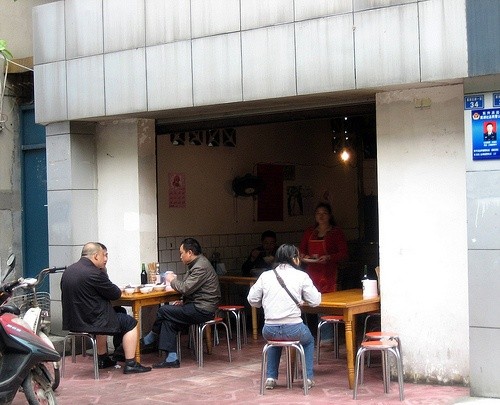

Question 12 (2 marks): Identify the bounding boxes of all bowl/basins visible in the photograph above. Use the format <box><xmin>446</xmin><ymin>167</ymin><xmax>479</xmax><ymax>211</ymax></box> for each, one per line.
<box><xmin>251</xmin><ymin>269</ymin><xmax>266</xmax><ymax>274</ymax></box>
<box><xmin>263</xmin><ymin>257</ymin><xmax>274</xmax><ymax>260</ymax></box>
<box><xmin>125</xmin><ymin>284</ymin><xmax>166</xmax><ymax>293</ymax></box>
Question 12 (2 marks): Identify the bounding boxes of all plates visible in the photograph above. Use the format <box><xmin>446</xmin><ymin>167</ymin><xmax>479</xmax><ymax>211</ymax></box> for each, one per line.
<box><xmin>302</xmin><ymin>257</ymin><xmax>321</xmax><ymax>262</ymax></box>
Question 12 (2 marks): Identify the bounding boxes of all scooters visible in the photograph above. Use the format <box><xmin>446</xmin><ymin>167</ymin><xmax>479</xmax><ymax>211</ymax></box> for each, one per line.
<box><xmin>0</xmin><ymin>253</ymin><xmax>61</xmax><ymax>405</ymax></box>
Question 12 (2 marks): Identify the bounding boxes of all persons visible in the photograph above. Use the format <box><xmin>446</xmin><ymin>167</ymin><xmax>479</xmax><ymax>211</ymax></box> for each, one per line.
<box><xmin>241</xmin><ymin>230</ymin><xmax>280</xmax><ymax>277</ymax></box>
<box><xmin>483</xmin><ymin>123</ymin><xmax>496</xmax><ymax>141</ymax></box>
<box><xmin>247</xmin><ymin>242</ymin><xmax>322</xmax><ymax>390</ymax></box>
<box><xmin>299</xmin><ymin>203</ymin><xmax>350</xmax><ymax>347</ymax></box>
<box><xmin>60</xmin><ymin>243</ymin><xmax>152</xmax><ymax>374</ymax></box>
<box><xmin>139</xmin><ymin>238</ymin><xmax>221</xmax><ymax>368</ymax></box>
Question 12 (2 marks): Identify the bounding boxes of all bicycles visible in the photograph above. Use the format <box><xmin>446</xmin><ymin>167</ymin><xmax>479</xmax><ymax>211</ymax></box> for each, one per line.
<box><xmin>10</xmin><ymin>265</ymin><xmax>67</xmax><ymax>391</ymax></box>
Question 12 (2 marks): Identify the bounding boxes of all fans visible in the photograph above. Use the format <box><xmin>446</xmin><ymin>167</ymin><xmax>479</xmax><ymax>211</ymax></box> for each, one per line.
<box><xmin>232</xmin><ymin>173</ymin><xmax>266</xmax><ymax>201</ymax></box>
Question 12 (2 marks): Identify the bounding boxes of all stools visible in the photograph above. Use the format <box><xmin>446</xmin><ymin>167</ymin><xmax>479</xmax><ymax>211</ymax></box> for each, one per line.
<box><xmin>61</xmin><ymin>305</ymin><xmax>405</xmax><ymax>401</ymax></box>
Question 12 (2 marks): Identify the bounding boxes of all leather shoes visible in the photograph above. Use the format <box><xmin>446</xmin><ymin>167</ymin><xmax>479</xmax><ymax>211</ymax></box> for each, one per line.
<box><xmin>123</xmin><ymin>362</ymin><xmax>152</xmax><ymax>373</ymax></box>
<box><xmin>153</xmin><ymin>358</ymin><xmax>180</xmax><ymax>368</ymax></box>
<box><xmin>139</xmin><ymin>338</ymin><xmax>155</xmax><ymax>349</ymax></box>
<box><xmin>98</xmin><ymin>359</ymin><xmax>116</xmax><ymax>369</ymax></box>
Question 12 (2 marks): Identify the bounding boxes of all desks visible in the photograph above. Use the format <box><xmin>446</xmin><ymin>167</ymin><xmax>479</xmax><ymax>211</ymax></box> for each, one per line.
<box><xmin>301</xmin><ymin>289</ymin><xmax>381</xmax><ymax>390</ymax></box>
<box><xmin>120</xmin><ymin>290</ymin><xmax>181</xmax><ymax>362</ymax></box>
<box><xmin>218</xmin><ymin>274</ymin><xmax>258</xmax><ymax>340</ymax></box>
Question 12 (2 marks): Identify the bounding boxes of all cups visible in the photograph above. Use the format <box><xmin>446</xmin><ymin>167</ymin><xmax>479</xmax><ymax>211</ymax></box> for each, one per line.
<box><xmin>361</xmin><ymin>280</ymin><xmax>378</xmax><ymax>299</ymax></box>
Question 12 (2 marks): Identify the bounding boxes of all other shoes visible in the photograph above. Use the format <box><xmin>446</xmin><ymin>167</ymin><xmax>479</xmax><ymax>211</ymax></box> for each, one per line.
<box><xmin>265</xmin><ymin>379</ymin><xmax>276</xmax><ymax>389</ymax></box>
<box><xmin>319</xmin><ymin>344</ymin><xmax>333</xmax><ymax>352</ymax></box>
<box><xmin>302</xmin><ymin>380</ymin><xmax>313</xmax><ymax>389</ymax></box>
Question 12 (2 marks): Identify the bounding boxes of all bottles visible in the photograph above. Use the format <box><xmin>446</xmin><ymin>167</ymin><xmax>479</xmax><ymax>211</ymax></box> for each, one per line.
<box><xmin>155</xmin><ymin>263</ymin><xmax>161</xmax><ymax>285</ymax></box>
<box><xmin>362</xmin><ymin>265</ymin><xmax>369</xmax><ymax>280</ymax></box>
<box><xmin>141</xmin><ymin>263</ymin><xmax>147</xmax><ymax>284</ymax></box>
<box><xmin>211</xmin><ymin>253</ymin><xmax>217</xmax><ymax>273</ymax></box>
